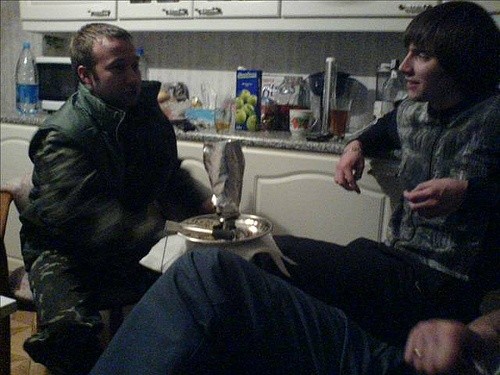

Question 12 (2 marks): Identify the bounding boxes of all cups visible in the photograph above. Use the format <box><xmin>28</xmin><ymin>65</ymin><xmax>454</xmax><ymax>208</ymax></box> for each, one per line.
<box><xmin>289</xmin><ymin>108</ymin><xmax>318</xmax><ymax>136</ymax></box>
<box><xmin>330</xmin><ymin>96</ymin><xmax>353</xmax><ymax>143</ymax></box>
<box><xmin>215</xmin><ymin>93</ymin><xmax>232</xmax><ymax>132</ymax></box>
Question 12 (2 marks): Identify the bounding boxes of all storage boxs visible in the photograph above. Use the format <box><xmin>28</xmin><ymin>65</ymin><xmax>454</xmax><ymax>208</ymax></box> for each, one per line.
<box><xmin>235</xmin><ymin>67</ymin><xmax>264</xmax><ymax>130</ymax></box>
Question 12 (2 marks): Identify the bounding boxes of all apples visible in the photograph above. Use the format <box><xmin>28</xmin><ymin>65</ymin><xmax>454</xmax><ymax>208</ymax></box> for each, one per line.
<box><xmin>234</xmin><ymin>90</ymin><xmax>259</xmax><ymax>132</ymax></box>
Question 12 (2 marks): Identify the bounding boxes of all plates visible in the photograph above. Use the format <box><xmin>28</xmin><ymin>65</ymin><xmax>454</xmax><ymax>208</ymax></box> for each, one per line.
<box><xmin>178</xmin><ymin>214</ymin><xmax>273</xmax><ymax>245</ymax></box>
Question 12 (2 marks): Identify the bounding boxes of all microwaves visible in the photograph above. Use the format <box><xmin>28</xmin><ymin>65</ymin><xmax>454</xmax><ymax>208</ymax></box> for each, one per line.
<box><xmin>34</xmin><ymin>53</ymin><xmax>79</xmax><ymax>111</ymax></box>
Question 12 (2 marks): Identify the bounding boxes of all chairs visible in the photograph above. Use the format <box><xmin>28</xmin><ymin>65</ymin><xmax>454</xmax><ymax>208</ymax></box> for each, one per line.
<box><xmin>0</xmin><ymin>173</ymin><xmax>122</xmax><ymax>375</ymax></box>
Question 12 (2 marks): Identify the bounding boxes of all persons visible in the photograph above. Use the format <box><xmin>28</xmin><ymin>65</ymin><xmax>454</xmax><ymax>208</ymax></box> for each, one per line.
<box><xmin>20</xmin><ymin>23</ymin><xmax>214</xmax><ymax>375</ymax></box>
<box><xmin>256</xmin><ymin>0</ymin><xmax>500</xmax><ymax>335</ymax></box>
<box><xmin>88</xmin><ymin>247</ymin><xmax>500</xmax><ymax>375</ymax></box>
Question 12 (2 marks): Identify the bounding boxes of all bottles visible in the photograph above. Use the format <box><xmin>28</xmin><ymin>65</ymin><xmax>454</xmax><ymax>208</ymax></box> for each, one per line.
<box><xmin>378</xmin><ymin>60</ymin><xmax>408</xmax><ymax>119</ymax></box>
<box><xmin>275</xmin><ymin>75</ymin><xmax>310</xmax><ymax>133</ymax></box>
<box><xmin>135</xmin><ymin>48</ymin><xmax>149</xmax><ymax>82</ymax></box>
<box><xmin>14</xmin><ymin>40</ymin><xmax>39</xmax><ymax>115</ymax></box>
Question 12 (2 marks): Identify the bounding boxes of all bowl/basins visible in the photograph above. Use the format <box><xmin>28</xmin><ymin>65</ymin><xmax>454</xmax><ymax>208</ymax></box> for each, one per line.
<box><xmin>308</xmin><ymin>71</ymin><xmax>350</xmax><ymax>96</ymax></box>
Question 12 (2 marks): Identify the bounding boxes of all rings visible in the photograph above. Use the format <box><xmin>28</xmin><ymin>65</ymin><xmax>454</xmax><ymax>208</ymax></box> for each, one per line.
<box><xmin>413</xmin><ymin>349</ymin><xmax>421</xmax><ymax>358</ymax></box>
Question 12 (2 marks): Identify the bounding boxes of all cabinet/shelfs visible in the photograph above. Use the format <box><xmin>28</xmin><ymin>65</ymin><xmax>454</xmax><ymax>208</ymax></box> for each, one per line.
<box><xmin>280</xmin><ymin>1</ymin><xmax>443</xmax><ymax>30</ymax></box>
<box><xmin>142</xmin><ymin>140</ymin><xmax>397</xmax><ymax>278</ymax></box>
<box><xmin>20</xmin><ymin>1</ymin><xmax>118</xmax><ymax>33</ymax></box>
<box><xmin>118</xmin><ymin>0</ymin><xmax>282</xmax><ymax>31</ymax></box>
